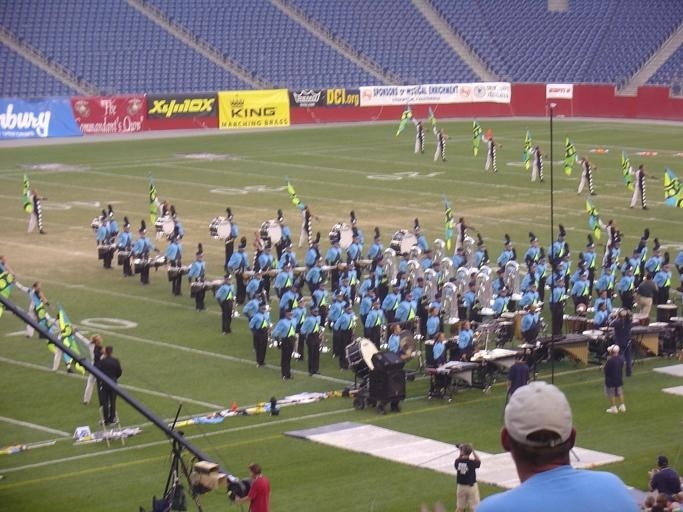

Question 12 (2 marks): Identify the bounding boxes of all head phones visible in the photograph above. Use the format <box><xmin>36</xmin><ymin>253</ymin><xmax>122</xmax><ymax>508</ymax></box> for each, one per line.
<box><xmin>618</xmin><ymin>308</ymin><xmax>624</xmax><ymax>317</ymax></box>
<box><xmin>609</xmin><ymin>345</ymin><xmax>616</xmax><ymax>353</ymax></box>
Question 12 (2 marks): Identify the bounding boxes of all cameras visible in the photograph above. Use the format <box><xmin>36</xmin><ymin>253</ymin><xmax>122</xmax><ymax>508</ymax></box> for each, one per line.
<box><xmin>455</xmin><ymin>442</ymin><xmax>462</xmax><ymax>451</ymax></box>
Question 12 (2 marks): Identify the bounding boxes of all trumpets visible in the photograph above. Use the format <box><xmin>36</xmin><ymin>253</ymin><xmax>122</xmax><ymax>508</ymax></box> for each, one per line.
<box><xmin>227</xmin><ymin>275</ymin><xmax>424</xmax><ymax>368</ymax></box>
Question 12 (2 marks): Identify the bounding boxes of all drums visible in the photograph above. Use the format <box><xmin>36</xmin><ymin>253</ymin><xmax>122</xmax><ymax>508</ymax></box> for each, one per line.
<box><xmin>260</xmin><ymin>219</ymin><xmax>282</xmax><ymax>249</ymax></box>
<box><xmin>329</xmin><ymin>224</ymin><xmax>354</xmax><ymax>248</ymax></box>
<box><xmin>154</xmin><ymin>216</ymin><xmax>174</xmax><ymax>237</ymax></box>
<box><xmin>390</xmin><ymin>231</ymin><xmax>417</xmax><ymax>256</ymax></box>
<box><xmin>90</xmin><ymin>215</ymin><xmax>225</xmax><ymax>296</ymax></box>
<box><xmin>344</xmin><ymin>336</ymin><xmax>377</xmax><ymax>376</ymax></box>
<box><xmin>208</xmin><ymin>215</ymin><xmax>231</xmax><ymax>240</ymax></box>
<box><xmin>570</xmin><ymin>295</ymin><xmax>682</xmax><ymax>360</ymax></box>
<box><xmin>425</xmin><ymin>338</ymin><xmax>448</xmax><ymax>363</ymax></box>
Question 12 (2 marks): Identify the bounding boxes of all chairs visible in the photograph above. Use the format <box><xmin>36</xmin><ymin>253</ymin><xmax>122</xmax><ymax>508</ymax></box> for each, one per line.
<box><xmin>0</xmin><ymin>0</ymin><xmax>683</xmax><ymax>97</ymax></box>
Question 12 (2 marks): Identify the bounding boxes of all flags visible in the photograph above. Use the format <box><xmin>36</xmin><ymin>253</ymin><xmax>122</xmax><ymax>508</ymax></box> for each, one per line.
<box><xmin>150</xmin><ymin>184</ymin><xmax>157</xmax><ymax>225</ymax></box>
<box><xmin>287</xmin><ymin>185</ymin><xmax>304</xmax><ymax>211</ymax></box>
<box><xmin>24</xmin><ymin>178</ymin><xmax>32</xmax><ymax>213</ymax></box>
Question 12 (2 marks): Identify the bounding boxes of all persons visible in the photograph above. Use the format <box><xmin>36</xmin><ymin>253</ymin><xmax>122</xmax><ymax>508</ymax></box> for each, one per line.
<box><xmin>27</xmin><ymin>189</ymin><xmax>48</xmax><ymax>234</ymax></box>
<box><xmin>455</xmin><ymin>444</ymin><xmax>481</xmax><ymax>512</ymax></box>
<box><xmin>643</xmin><ymin>456</ymin><xmax>682</xmax><ymax>512</ymax></box>
<box><xmin>481</xmin><ymin>134</ymin><xmax>497</xmax><ymax>173</ymax></box>
<box><xmin>433</xmin><ymin>125</ymin><xmax>450</xmax><ymax>161</ymax></box>
<box><xmin>0</xmin><ymin>256</ymin><xmax>122</xmax><ymax>425</ymax></box>
<box><xmin>528</xmin><ymin>145</ymin><xmax>544</xmax><ymax>184</ymax></box>
<box><xmin>575</xmin><ymin>154</ymin><xmax>597</xmax><ymax>195</ymax></box>
<box><xmin>628</xmin><ymin>164</ymin><xmax>649</xmax><ymax>209</ymax></box>
<box><xmin>412</xmin><ymin>117</ymin><xmax>425</xmax><ymax>155</ymax></box>
<box><xmin>188</xmin><ymin>251</ymin><xmax>206</xmax><ymax>311</ymax></box>
<box><xmin>475</xmin><ymin>381</ymin><xmax>642</xmax><ymax>512</ymax></box>
<box><xmin>235</xmin><ymin>464</ymin><xmax>271</xmax><ymax>512</ymax></box>
<box><xmin>97</xmin><ymin>197</ymin><xmax>184</xmax><ymax>296</ymax></box>
<box><xmin>603</xmin><ymin>344</ymin><xmax>626</xmax><ymax>414</ymax></box>
<box><xmin>215</xmin><ymin>204</ymin><xmax>682</xmax><ymax>391</ymax></box>
<box><xmin>507</xmin><ymin>352</ymin><xmax>529</xmax><ymax>401</ymax></box>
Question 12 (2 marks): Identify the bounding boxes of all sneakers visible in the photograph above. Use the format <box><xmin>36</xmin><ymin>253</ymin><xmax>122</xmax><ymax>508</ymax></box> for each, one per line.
<box><xmin>619</xmin><ymin>404</ymin><xmax>626</xmax><ymax>413</ymax></box>
<box><xmin>606</xmin><ymin>406</ymin><xmax>617</xmax><ymax>414</ymax></box>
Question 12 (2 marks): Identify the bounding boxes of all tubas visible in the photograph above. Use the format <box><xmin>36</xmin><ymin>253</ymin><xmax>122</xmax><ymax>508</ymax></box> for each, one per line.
<box><xmin>378</xmin><ymin>235</ymin><xmax>525</xmax><ymax>328</ymax></box>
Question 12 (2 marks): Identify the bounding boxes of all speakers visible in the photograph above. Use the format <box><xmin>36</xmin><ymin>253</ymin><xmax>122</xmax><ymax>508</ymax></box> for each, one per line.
<box><xmin>370</xmin><ymin>370</ymin><xmax>406</xmax><ymax>400</ymax></box>
<box><xmin>657</xmin><ymin>304</ymin><xmax>677</xmax><ymax>353</ymax></box>
<box><xmin>371</xmin><ymin>351</ymin><xmax>405</xmax><ymax>373</ymax></box>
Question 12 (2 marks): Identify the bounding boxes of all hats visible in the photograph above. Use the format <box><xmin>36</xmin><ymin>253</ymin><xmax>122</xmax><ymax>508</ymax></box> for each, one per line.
<box><xmin>657</xmin><ymin>455</ymin><xmax>668</xmax><ymax>465</ymax></box>
<box><xmin>504</xmin><ymin>380</ymin><xmax>572</xmax><ymax>447</ymax></box>
<box><xmin>607</xmin><ymin>344</ymin><xmax>620</xmax><ymax>353</ymax></box>
